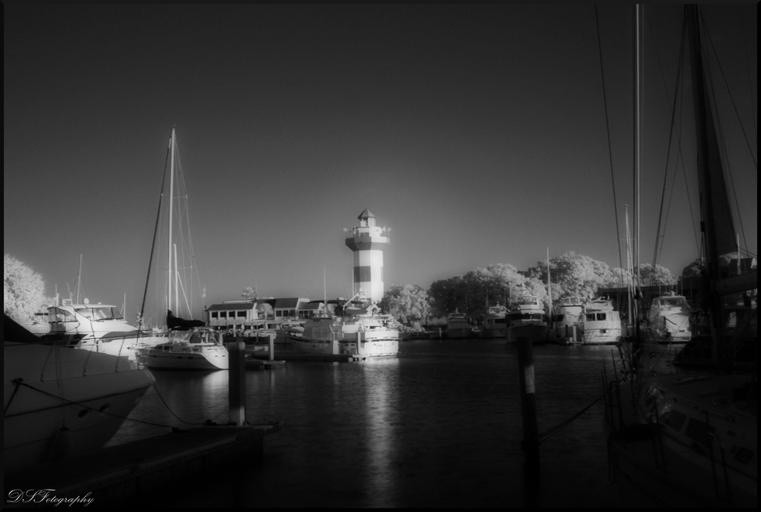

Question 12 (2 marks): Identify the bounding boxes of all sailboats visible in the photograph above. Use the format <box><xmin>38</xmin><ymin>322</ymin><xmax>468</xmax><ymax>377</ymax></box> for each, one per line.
<box><xmin>630</xmin><ymin>2</ymin><xmax>761</xmax><ymax>504</ymax></box>
<box><xmin>136</xmin><ymin>126</ymin><xmax>252</xmax><ymax>370</ymax></box>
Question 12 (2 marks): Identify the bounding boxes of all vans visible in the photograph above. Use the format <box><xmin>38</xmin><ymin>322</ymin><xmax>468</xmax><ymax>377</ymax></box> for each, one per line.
<box><xmin>229</xmin><ymin>329</ymin><xmax>276</xmax><ymax>337</ymax></box>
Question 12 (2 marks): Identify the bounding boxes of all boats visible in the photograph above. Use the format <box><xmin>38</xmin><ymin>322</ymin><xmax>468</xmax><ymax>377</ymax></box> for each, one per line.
<box><xmin>4</xmin><ymin>283</ymin><xmax>169</xmax><ymax>420</ymax></box>
<box><xmin>287</xmin><ymin>314</ymin><xmax>400</xmax><ymax>358</ymax></box>
<box><xmin>446</xmin><ymin>281</ymin><xmax>701</xmax><ymax>342</ymax></box>
<box><xmin>4</xmin><ymin>414</ymin><xmax>281</xmax><ymax>511</ymax></box>
<box><xmin>647</xmin><ymin>342</ymin><xmax>694</xmax><ymax>374</ymax></box>
